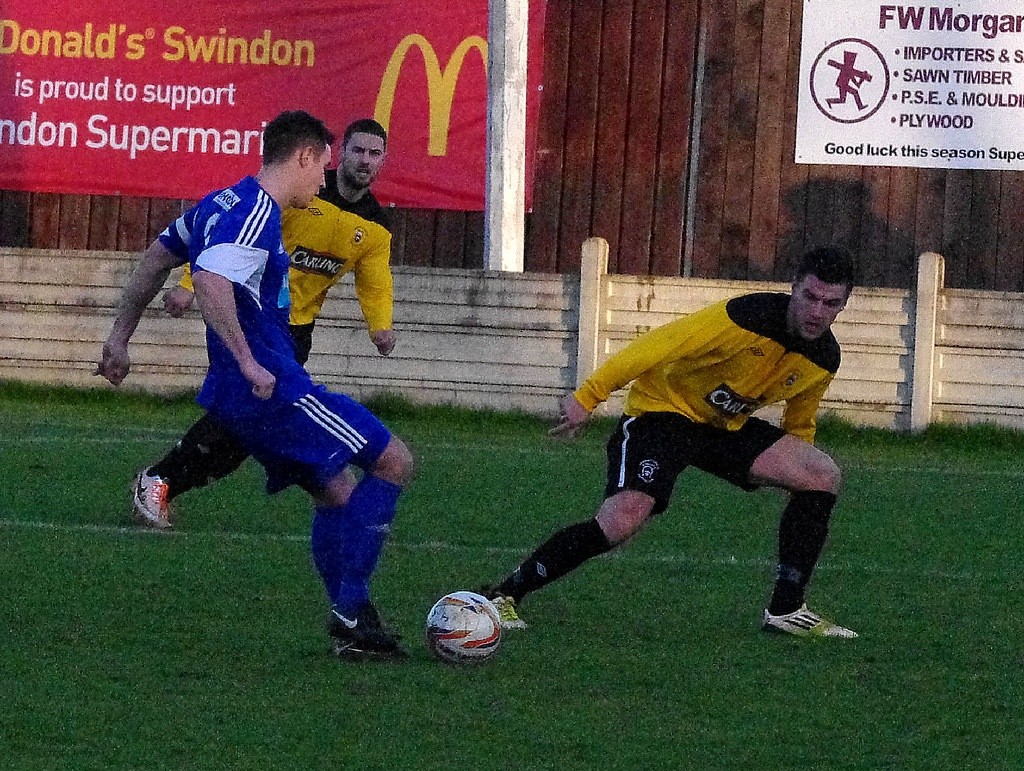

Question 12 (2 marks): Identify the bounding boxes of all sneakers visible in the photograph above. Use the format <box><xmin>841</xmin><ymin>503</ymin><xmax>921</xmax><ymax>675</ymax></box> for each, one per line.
<box><xmin>326</xmin><ymin>598</ymin><xmax>398</xmax><ymax>652</ymax></box>
<box><xmin>336</xmin><ymin>640</ymin><xmax>413</xmax><ymax>665</ymax></box>
<box><xmin>493</xmin><ymin>594</ymin><xmax>528</xmax><ymax>628</ymax></box>
<box><xmin>759</xmin><ymin>603</ymin><xmax>860</xmax><ymax>639</ymax></box>
<box><xmin>131</xmin><ymin>466</ymin><xmax>170</xmax><ymax>526</ymax></box>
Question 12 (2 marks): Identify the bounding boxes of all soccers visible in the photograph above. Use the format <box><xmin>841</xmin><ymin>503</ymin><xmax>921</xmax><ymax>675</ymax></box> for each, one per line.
<box><xmin>426</xmin><ymin>591</ymin><xmax>502</xmax><ymax>661</ymax></box>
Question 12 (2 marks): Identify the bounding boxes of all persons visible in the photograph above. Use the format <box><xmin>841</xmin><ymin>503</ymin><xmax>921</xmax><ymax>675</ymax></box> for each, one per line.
<box><xmin>132</xmin><ymin>118</ymin><xmax>396</xmax><ymax>526</ymax></box>
<box><xmin>93</xmin><ymin>110</ymin><xmax>412</xmax><ymax>663</ymax></box>
<box><xmin>487</xmin><ymin>247</ymin><xmax>860</xmax><ymax>640</ymax></box>
